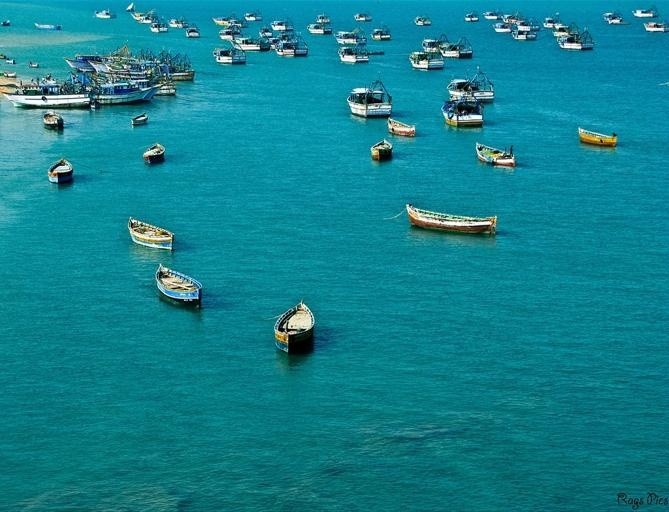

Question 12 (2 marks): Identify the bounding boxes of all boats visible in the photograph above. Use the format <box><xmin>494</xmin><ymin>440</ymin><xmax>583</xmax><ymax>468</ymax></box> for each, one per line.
<box><xmin>155</xmin><ymin>265</ymin><xmax>202</xmax><ymax>310</ymax></box>
<box><xmin>143</xmin><ymin>144</ymin><xmax>166</xmax><ymax>165</ymax></box>
<box><xmin>48</xmin><ymin>159</ymin><xmax>73</xmax><ymax>185</ymax></box>
<box><xmin>577</xmin><ymin>126</ymin><xmax>620</xmax><ymax>148</ymax></box>
<box><xmin>0</xmin><ymin>1</ymin><xmax>669</xmax><ymax>135</ymax></box>
<box><xmin>128</xmin><ymin>218</ymin><xmax>174</xmax><ymax>253</ymax></box>
<box><xmin>476</xmin><ymin>142</ymin><xmax>515</xmax><ymax>167</ymax></box>
<box><xmin>272</xmin><ymin>300</ymin><xmax>315</xmax><ymax>354</ymax></box>
<box><xmin>371</xmin><ymin>140</ymin><xmax>391</xmax><ymax>161</ymax></box>
<box><xmin>405</xmin><ymin>203</ymin><xmax>497</xmax><ymax>236</ymax></box>
